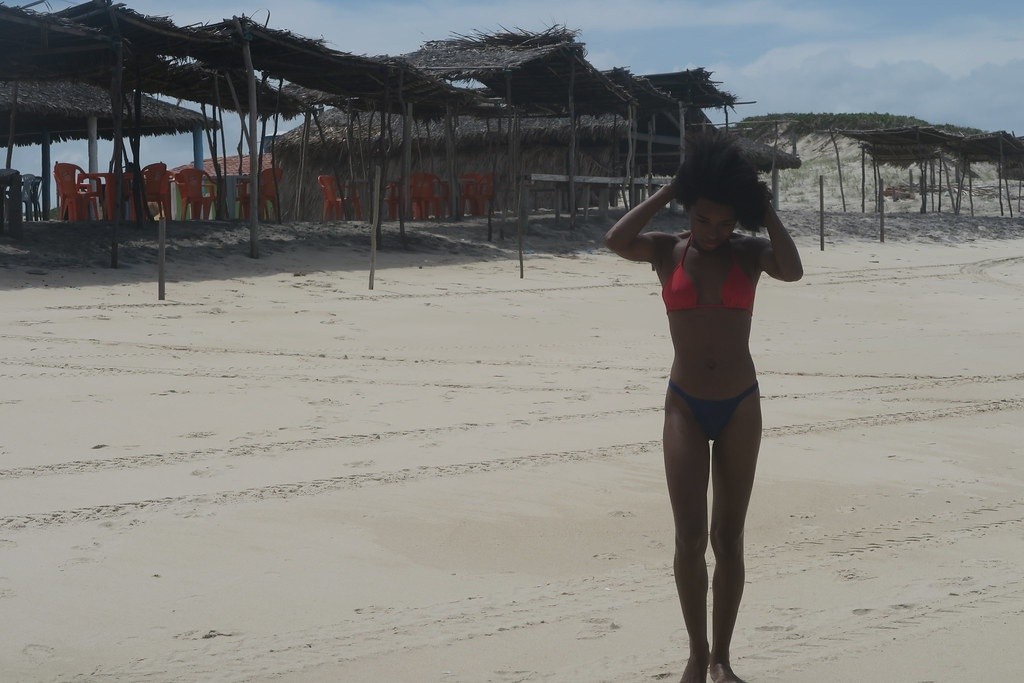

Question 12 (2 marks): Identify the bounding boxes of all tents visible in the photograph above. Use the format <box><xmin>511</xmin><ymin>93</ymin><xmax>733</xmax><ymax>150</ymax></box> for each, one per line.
<box><xmin>1</xmin><ymin>0</ymin><xmax>800</xmax><ymax>260</ymax></box>
<box><xmin>824</xmin><ymin>125</ymin><xmax>1024</xmax><ymax>218</ymax></box>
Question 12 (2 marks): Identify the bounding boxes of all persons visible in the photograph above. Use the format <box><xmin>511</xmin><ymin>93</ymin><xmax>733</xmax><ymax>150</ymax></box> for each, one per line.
<box><xmin>604</xmin><ymin>132</ymin><xmax>803</xmax><ymax>683</ymax></box>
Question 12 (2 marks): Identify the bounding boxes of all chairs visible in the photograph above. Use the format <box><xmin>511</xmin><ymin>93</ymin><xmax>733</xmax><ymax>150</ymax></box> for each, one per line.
<box><xmin>0</xmin><ymin>164</ymin><xmax>492</xmax><ymax>222</ymax></box>
